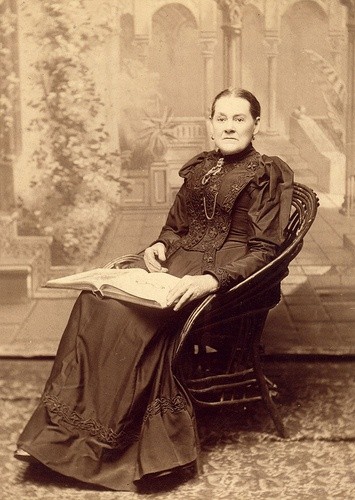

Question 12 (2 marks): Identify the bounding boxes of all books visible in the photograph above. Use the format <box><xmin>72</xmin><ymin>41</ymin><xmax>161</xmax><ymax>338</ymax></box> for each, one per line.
<box><xmin>42</xmin><ymin>268</ymin><xmax>184</xmax><ymax>310</ymax></box>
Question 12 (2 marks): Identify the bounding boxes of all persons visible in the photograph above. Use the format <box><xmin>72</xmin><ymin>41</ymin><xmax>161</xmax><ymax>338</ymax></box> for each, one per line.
<box><xmin>14</xmin><ymin>88</ymin><xmax>294</xmax><ymax>491</ymax></box>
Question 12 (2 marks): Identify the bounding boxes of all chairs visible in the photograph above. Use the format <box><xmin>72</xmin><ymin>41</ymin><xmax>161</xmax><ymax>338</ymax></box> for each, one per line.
<box><xmin>171</xmin><ymin>181</ymin><xmax>321</xmax><ymax>443</ymax></box>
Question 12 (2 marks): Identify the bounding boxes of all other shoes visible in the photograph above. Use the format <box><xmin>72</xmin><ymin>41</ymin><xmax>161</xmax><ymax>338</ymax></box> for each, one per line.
<box><xmin>13</xmin><ymin>448</ymin><xmax>40</xmax><ymax>462</ymax></box>
<box><xmin>154</xmin><ymin>463</ymin><xmax>195</xmax><ymax>481</ymax></box>
